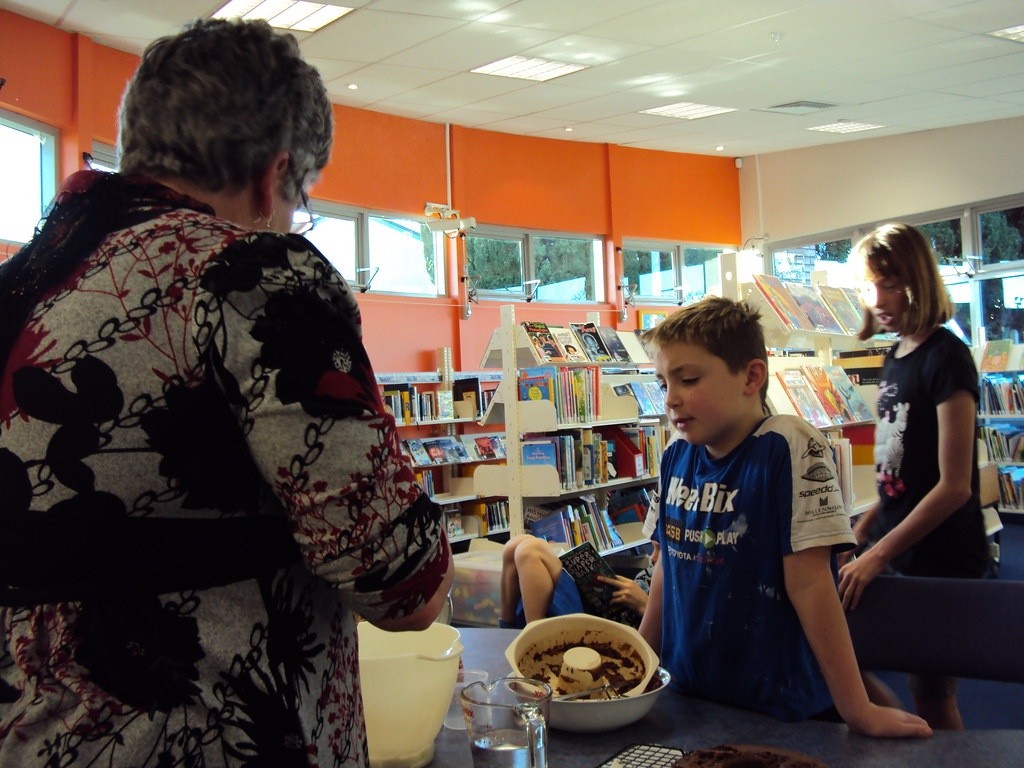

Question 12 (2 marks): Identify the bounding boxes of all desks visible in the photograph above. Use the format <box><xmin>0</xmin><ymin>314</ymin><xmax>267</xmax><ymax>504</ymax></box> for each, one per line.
<box><xmin>425</xmin><ymin>624</ymin><xmax>1024</xmax><ymax>768</ymax></box>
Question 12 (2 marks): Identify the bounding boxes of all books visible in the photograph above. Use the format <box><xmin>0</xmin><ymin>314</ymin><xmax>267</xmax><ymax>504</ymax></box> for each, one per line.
<box><xmin>974</xmin><ymin>338</ymin><xmax>1024</xmax><ymax>510</ymax></box>
<box><xmin>558</xmin><ymin>542</ymin><xmax>623</xmax><ymax>613</ymax></box>
<box><xmin>752</xmin><ymin>273</ymin><xmax>902</xmax><ymax>512</ymax></box>
<box><xmin>380</xmin><ymin>320</ymin><xmax>677</xmax><ymax>553</ymax></box>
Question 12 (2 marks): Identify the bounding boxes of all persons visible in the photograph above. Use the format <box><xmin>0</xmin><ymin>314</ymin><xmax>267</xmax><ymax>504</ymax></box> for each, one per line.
<box><xmin>547</xmin><ymin>334</ymin><xmax>563</xmax><ymax>357</ymax></box>
<box><xmin>1</xmin><ymin>16</ymin><xmax>456</xmax><ymax>768</ymax></box>
<box><xmin>537</xmin><ymin>332</ymin><xmax>558</xmax><ymax>357</ymax></box>
<box><xmin>836</xmin><ymin>223</ymin><xmax>999</xmax><ymax>734</ymax></box>
<box><xmin>638</xmin><ymin>298</ymin><xmax>933</xmax><ymax>737</ymax></box>
<box><xmin>530</xmin><ymin>336</ymin><xmax>549</xmax><ymax>358</ymax></box>
<box><xmin>564</xmin><ymin>344</ymin><xmax>585</xmax><ymax>361</ymax></box>
<box><xmin>497</xmin><ymin>519</ymin><xmax>661</xmax><ymax>630</ymax></box>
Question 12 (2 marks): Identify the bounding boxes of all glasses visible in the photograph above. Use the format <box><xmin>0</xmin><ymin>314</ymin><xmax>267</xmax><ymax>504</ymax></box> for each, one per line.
<box><xmin>288</xmin><ymin>154</ymin><xmax>324</xmax><ymax>236</ymax></box>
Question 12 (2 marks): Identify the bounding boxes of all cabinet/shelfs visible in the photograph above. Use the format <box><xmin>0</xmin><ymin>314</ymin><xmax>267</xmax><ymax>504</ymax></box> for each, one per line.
<box><xmin>371</xmin><ymin>284</ymin><xmax>1024</xmax><ymax>567</ymax></box>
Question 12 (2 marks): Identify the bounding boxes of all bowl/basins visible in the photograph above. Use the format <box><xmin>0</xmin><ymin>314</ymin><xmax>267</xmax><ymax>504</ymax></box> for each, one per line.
<box><xmin>504</xmin><ymin>668</ymin><xmax>671</xmax><ymax>731</ymax></box>
<box><xmin>357</xmin><ymin>620</ymin><xmax>462</xmax><ymax>768</ymax></box>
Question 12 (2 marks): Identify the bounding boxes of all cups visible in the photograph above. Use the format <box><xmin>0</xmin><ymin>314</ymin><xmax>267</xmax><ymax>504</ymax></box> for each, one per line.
<box><xmin>460</xmin><ymin>676</ymin><xmax>553</xmax><ymax>768</ymax></box>
<box><xmin>443</xmin><ymin>669</ymin><xmax>492</xmax><ymax>730</ymax></box>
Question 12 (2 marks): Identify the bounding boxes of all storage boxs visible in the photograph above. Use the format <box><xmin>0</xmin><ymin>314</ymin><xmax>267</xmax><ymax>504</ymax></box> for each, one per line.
<box><xmin>451</xmin><ymin>551</ymin><xmax>503</xmax><ymax>629</ymax></box>
